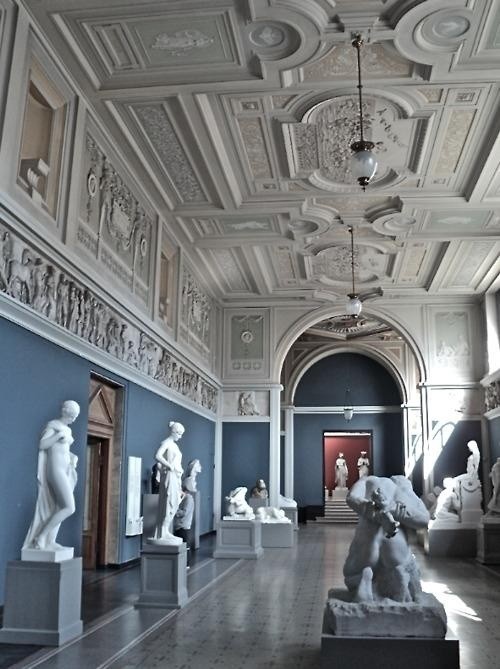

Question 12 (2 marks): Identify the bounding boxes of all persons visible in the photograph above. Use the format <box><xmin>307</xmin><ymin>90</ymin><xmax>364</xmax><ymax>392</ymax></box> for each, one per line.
<box><xmin>435</xmin><ymin>477</ymin><xmax>463</xmax><ymax>523</ymax></box>
<box><xmin>448</xmin><ymin>333</ymin><xmax>469</xmax><ymax>357</ymax></box>
<box><xmin>182</xmin><ymin>458</ymin><xmax>203</xmax><ymax>493</ymax></box>
<box><xmin>172</xmin><ymin>487</ymin><xmax>194</xmax><ymax>571</ymax></box>
<box><xmin>151</xmin><ymin>420</ymin><xmax>187</xmax><ymax>543</ymax></box>
<box><xmin>437</xmin><ymin>339</ymin><xmax>456</xmax><ymax>357</ymax></box>
<box><xmin>339</xmin><ymin>474</ymin><xmax>431</xmax><ymax>607</ymax></box>
<box><xmin>334</xmin><ymin>452</ymin><xmax>349</xmax><ymax>490</ymax></box>
<box><xmin>21</xmin><ymin>398</ymin><xmax>82</xmax><ymax>552</ymax></box>
<box><xmin>0</xmin><ymin>232</ymin><xmax>220</xmax><ymax>415</ymax></box>
<box><xmin>356</xmin><ymin>449</ymin><xmax>371</xmax><ymax>478</ymax></box>
<box><xmin>244</xmin><ymin>393</ymin><xmax>261</xmax><ymax>416</ymax></box>
<box><xmin>452</xmin><ymin>438</ymin><xmax>481</xmax><ymax>492</ymax></box>
<box><xmin>237</xmin><ymin>392</ymin><xmax>250</xmax><ymax>417</ymax></box>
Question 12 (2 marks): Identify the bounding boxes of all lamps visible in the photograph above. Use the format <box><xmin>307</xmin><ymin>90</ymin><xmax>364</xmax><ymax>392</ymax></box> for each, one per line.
<box><xmin>340</xmin><ymin>224</ymin><xmax>365</xmax><ymax>320</ymax></box>
<box><xmin>344</xmin><ymin>37</ymin><xmax>382</xmax><ymax>195</ymax></box>
<box><xmin>340</xmin><ymin>387</ymin><xmax>359</xmax><ymax>421</ymax></box>
